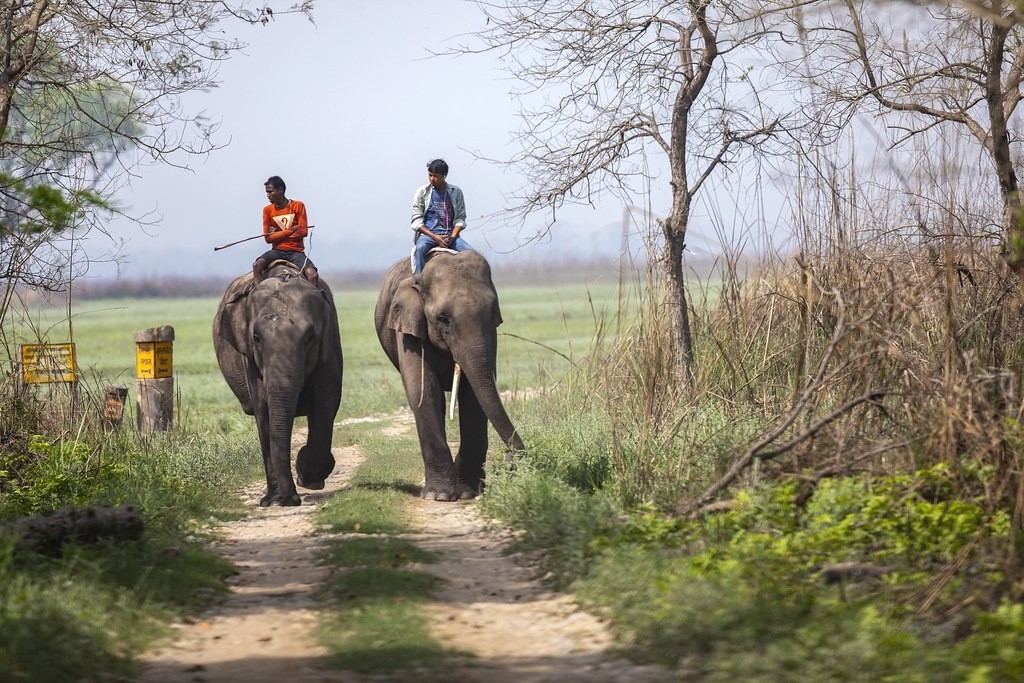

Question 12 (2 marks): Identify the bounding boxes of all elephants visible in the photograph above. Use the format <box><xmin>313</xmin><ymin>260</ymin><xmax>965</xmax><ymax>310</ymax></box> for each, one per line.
<box><xmin>212</xmin><ymin>262</ymin><xmax>343</xmax><ymax>507</ymax></box>
<box><xmin>375</xmin><ymin>247</ymin><xmax>527</xmax><ymax>502</ymax></box>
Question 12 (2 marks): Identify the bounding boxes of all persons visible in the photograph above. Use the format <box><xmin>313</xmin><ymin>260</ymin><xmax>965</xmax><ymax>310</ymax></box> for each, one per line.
<box><xmin>409</xmin><ymin>159</ymin><xmax>476</xmax><ymax>275</ymax></box>
<box><xmin>252</xmin><ymin>175</ymin><xmax>319</xmax><ymax>289</ymax></box>
